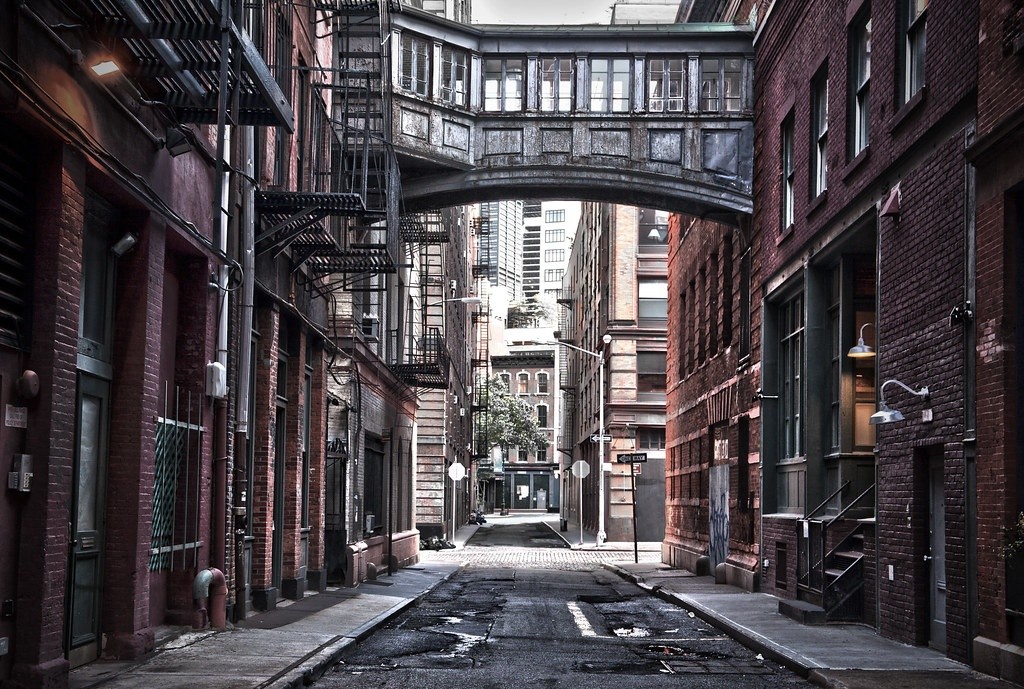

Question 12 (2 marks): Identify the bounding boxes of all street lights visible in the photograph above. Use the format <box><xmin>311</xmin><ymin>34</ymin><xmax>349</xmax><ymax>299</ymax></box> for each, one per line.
<box><xmin>548</xmin><ymin>339</ymin><xmax>607</xmax><ymax>546</ymax></box>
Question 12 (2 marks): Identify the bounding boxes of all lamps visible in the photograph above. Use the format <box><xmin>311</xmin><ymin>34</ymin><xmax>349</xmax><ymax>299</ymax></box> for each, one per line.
<box><xmin>160</xmin><ymin>125</ymin><xmax>193</xmax><ymax>160</ymax></box>
<box><xmin>74</xmin><ymin>50</ymin><xmax>120</xmax><ymax>76</ymax></box>
<box><xmin>848</xmin><ymin>321</ymin><xmax>874</xmax><ymax>358</ymax></box>
<box><xmin>869</xmin><ymin>379</ymin><xmax>928</xmax><ymax>426</ymax></box>
<box><xmin>103</xmin><ymin>224</ymin><xmax>142</xmax><ymax>256</ymax></box>
